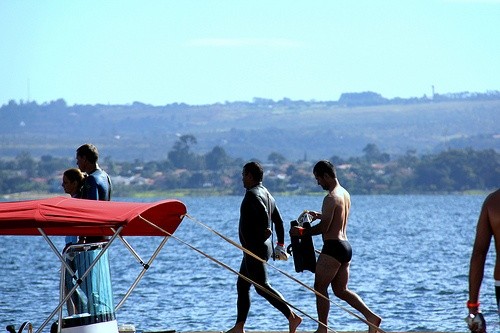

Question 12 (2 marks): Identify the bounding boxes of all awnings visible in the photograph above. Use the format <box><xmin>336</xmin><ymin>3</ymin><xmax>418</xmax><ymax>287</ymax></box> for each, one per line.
<box><xmin>0</xmin><ymin>196</ymin><xmax>187</xmax><ymax>333</ymax></box>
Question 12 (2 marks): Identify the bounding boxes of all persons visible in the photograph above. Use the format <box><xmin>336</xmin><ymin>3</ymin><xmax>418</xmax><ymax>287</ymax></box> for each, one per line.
<box><xmin>59</xmin><ymin>144</ymin><xmax>113</xmax><ymax>316</ymax></box>
<box><xmin>220</xmin><ymin>161</ymin><xmax>303</xmax><ymax>333</ymax></box>
<box><xmin>302</xmin><ymin>160</ymin><xmax>382</xmax><ymax>333</ymax></box>
<box><xmin>466</xmin><ymin>189</ymin><xmax>500</xmax><ymax>333</ymax></box>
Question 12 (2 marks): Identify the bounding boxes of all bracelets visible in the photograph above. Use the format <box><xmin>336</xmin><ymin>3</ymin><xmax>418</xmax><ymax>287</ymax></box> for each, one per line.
<box><xmin>467</xmin><ymin>301</ymin><xmax>480</xmax><ymax>308</ymax></box>
